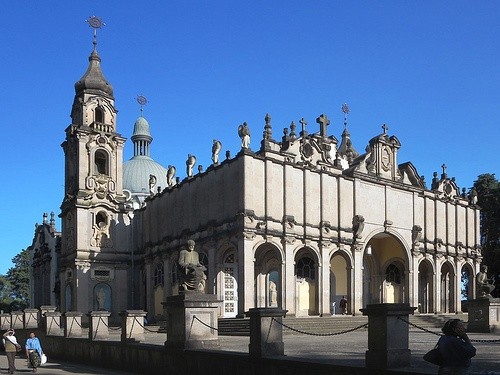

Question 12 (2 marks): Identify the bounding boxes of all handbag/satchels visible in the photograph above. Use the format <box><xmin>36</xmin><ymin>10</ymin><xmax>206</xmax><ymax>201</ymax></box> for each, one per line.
<box><xmin>42</xmin><ymin>354</ymin><xmax>47</xmax><ymax>364</ymax></box>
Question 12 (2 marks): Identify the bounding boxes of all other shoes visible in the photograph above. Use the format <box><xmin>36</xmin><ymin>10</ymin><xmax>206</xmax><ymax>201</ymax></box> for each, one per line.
<box><xmin>8</xmin><ymin>368</ymin><xmax>10</xmax><ymax>373</ymax></box>
<box><xmin>11</xmin><ymin>371</ymin><xmax>14</xmax><ymax>374</ymax></box>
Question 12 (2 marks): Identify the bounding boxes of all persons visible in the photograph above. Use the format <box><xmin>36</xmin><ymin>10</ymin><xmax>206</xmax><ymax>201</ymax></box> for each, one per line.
<box><xmin>211</xmin><ymin>139</ymin><xmax>222</xmax><ymax>164</ymax></box>
<box><xmin>269</xmin><ymin>275</ymin><xmax>278</xmax><ymax>302</ymax></box>
<box><xmin>167</xmin><ymin>165</ymin><xmax>176</xmax><ymax>186</ymax></box>
<box><xmin>241</xmin><ymin>122</ymin><xmax>250</xmax><ymax>149</ymax></box>
<box><xmin>97</xmin><ymin>287</ymin><xmax>105</xmax><ymax>309</ymax></box>
<box><xmin>25</xmin><ymin>332</ymin><xmax>43</xmax><ymax>373</ymax></box>
<box><xmin>186</xmin><ymin>154</ymin><xmax>196</xmax><ymax>176</ymax></box>
<box><xmin>3</xmin><ymin>329</ymin><xmax>17</xmax><ymax>374</ymax></box>
<box><xmin>476</xmin><ymin>266</ymin><xmax>495</xmax><ymax>297</ymax></box>
<box><xmin>178</xmin><ymin>240</ymin><xmax>207</xmax><ymax>291</ymax></box>
<box><xmin>434</xmin><ymin>319</ymin><xmax>476</xmax><ymax>375</ymax></box>
<box><xmin>149</xmin><ymin>174</ymin><xmax>157</xmax><ymax>195</ymax></box>
<box><xmin>340</xmin><ymin>296</ymin><xmax>347</xmax><ymax>315</ymax></box>
